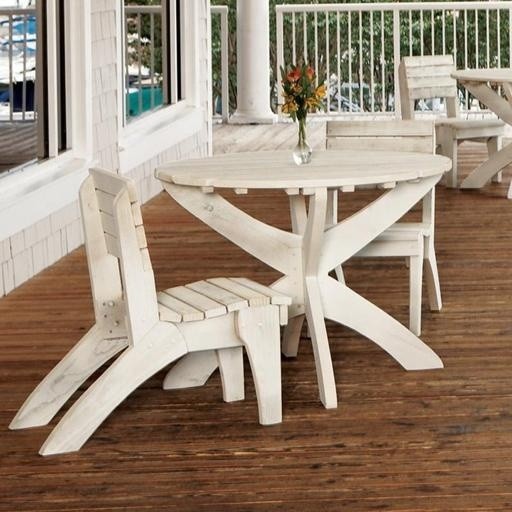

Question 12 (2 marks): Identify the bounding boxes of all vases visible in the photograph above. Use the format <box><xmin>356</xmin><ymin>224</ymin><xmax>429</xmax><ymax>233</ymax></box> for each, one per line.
<box><xmin>291</xmin><ymin>118</ymin><xmax>312</xmax><ymax>166</ymax></box>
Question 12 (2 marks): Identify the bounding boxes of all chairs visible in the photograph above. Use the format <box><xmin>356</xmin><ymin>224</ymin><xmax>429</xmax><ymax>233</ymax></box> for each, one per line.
<box><xmin>282</xmin><ymin>120</ymin><xmax>443</xmax><ymax>359</ymax></box>
<box><xmin>8</xmin><ymin>168</ymin><xmax>292</xmax><ymax>456</ymax></box>
<box><xmin>398</xmin><ymin>54</ymin><xmax>505</xmax><ymax>189</ymax></box>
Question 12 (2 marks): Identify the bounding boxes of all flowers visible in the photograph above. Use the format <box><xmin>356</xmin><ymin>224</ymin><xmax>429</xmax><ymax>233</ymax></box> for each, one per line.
<box><xmin>279</xmin><ymin>58</ymin><xmax>328</xmax><ymax>122</ymax></box>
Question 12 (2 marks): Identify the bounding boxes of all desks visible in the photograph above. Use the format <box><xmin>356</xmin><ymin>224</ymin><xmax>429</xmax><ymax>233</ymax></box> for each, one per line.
<box><xmin>450</xmin><ymin>70</ymin><xmax>512</xmax><ymax>199</ymax></box>
<box><xmin>153</xmin><ymin>151</ymin><xmax>453</xmax><ymax>391</ymax></box>
